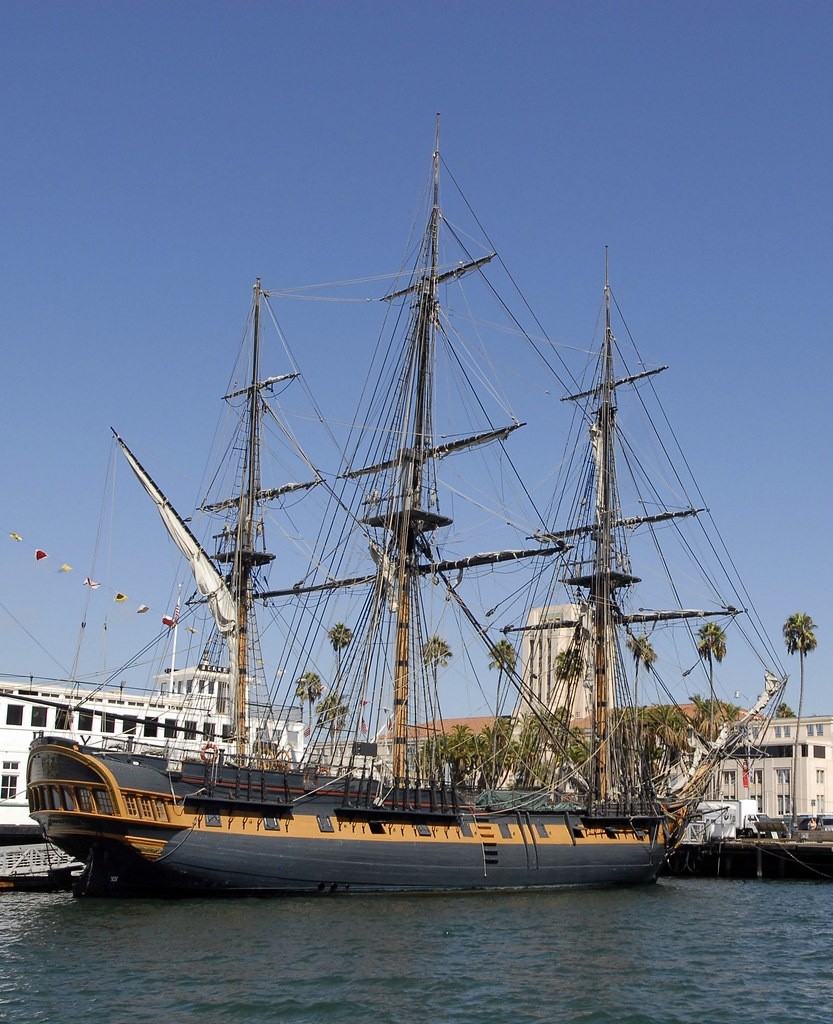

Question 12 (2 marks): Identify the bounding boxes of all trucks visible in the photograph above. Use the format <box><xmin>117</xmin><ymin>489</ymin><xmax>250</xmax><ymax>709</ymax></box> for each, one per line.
<box><xmin>697</xmin><ymin>799</ymin><xmax>772</xmax><ymax>838</ymax></box>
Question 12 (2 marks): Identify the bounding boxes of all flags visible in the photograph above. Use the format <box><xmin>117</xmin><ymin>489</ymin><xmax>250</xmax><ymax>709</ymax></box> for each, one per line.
<box><xmin>35</xmin><ymin>549</ymin><xmax>47</xmax><ymax>563</ymax></box>
<box><xmin>162</xmin><ymin>614</ymin><xmax>173</xmax><ymax>626</ymax></box>
<box><xmin>58</xmin><ymin>563</ymin><xmax>72</xmax><ymax>573</ymax></box>
<box><xmin>137</xmin><ymin>604</ymin><xmax>149</xmax><ymax>615</ymax></box>
<box><xmin>82</xmin><ymin>577</ymin><xmax>100</xmax><ymax>589</ymax></box>
<box><xmin>168</xmin><ymin>596</ymin><xmax>179</xmax><ymax>633</ymax></box>
<box><xmin>185</xmin><ymin>626</ymin><xmax>200</xmax><ymax>634</ymax></box>
<box><xmin>113</xmin><ymin>592</ymin><xmax>129</xmax><ymax>603</ymax></box>
<box><xmin>10</xmin><ymin>533</ymin><xmax>23</xmax><ymax>543</ymax></box>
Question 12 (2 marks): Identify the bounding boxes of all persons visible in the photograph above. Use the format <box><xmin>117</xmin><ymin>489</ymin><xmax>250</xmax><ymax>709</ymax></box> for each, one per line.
<box><xmin>807</xmin><ymin>816</ymin><xmax>823</xmax><ymax>832</ymax></box>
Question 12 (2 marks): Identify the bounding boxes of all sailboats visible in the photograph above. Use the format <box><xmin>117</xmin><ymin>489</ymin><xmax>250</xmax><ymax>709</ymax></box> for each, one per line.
<box><xmin>1</xmin><ymin>113</ymin><xmax>792</xmax><ymax>902</ymax></box>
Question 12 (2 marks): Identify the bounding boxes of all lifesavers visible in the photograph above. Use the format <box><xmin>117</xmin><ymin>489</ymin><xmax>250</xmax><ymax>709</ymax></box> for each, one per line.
<box><xmin>314</xmin><ymin>767</ymin><xmax>332</xmax><ymax>775</ymax></box>
<box><xmin>201</xmin><ymin>743</ymin><xmax>220</xmax><ymax>762</ymax></box>
